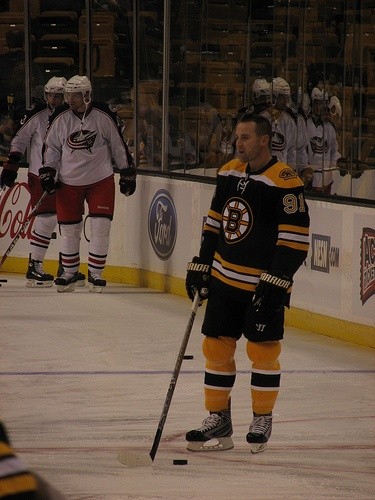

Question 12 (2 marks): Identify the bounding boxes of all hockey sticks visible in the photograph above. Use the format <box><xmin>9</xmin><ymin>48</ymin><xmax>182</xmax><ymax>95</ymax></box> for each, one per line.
<box><xmin>115</xmin><ymin>287</ymin><xmax>202</xmax><ymax>465</ymax></box>
<box><xmin>0</xmin><ymin>185</ymin><xmax>50</xmax><ymax>287</ymax></box>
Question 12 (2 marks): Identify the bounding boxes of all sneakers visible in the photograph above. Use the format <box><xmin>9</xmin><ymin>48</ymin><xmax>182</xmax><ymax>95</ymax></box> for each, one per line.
<box><xmin>57</xmin><ymin>267</ymin><xmax>86</xmax><ymax>286</ymax></box>
<box><xmin>246</xmin><ymin>411</ymin><xmax>272</xmax><ymax>454</ymax></box>
<box><xmin>26</xmin><ymin>259</ymin><xmax>54</xmax><ymax>287</ymax></box>
<box><xmin>87</xmin><ymin>269</ymin><xmax>106</xmax><ymax>293</ymax></box>
<box><xmin>185</xmin><ymin>398</ymin><xmax>234</xmax><ymax>452</ymax></box>
<box><xmin>55</xmin><ymin>271</ymin><xmax>79</xmax><ymax>293</ymax></box>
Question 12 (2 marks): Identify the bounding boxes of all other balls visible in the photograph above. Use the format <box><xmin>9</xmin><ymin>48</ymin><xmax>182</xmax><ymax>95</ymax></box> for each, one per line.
<box><xmin>0</xmin><ymin>279</ymin><xmax>7</xmax><ymax>283</ymax></box>
<box><xmin>183</xmin><ymin>356</ymin><xmax>193</xmax><ymax>359</ymax></box>
<box><xmin>173</xmin><ymin>460</ymin><xmax>188</xmax><ymax>464</ymax></box>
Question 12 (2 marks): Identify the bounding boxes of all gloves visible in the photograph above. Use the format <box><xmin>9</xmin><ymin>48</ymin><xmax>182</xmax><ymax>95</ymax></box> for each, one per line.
<box><xmin>118</xmin><ymin>167</ymin><xmax>136</xmax><ymax>197</ymax></box>
<box><xmin>39</xmin><ymin>166</ymin><xmax>57</xmax><ymax>194</ymax></box>
<box><xmin>186</xmin><ymin>256</ymin><xmax>212</xmax><ymax>306</ymax></box>
<box><xmin>337</xmin><ymin>157</ymin><xmax>347</xmax><ymax>177</ymax></box>
<box><xmin>252</xmin><ymin>272</ymin><xmax>293</xmax><ymax>315</ymax></box>
<box><xmin>0</xmin><ymin>151</ymin><xmax>22</xmax><ymax>189</ymax></box>
<box><xmin>304</xmin><ymin>168</ymin><xmax>314</xmax><ymax>191</ymax></box>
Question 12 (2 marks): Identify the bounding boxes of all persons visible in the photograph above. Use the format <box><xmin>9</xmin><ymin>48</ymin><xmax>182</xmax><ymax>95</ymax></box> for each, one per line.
<box><xmin>185</xmin><ymin>114</ymin><xmax>311</xmax><ymax>444</ymax></box>
<box><xmin>39</xmin><ymin>76</ymin><xmax>137</xmax><ymax>286</ymax></box>
<box><xmin>0</xmin><ymin>76</ymin><xmax>71</xmax><ymax>281</ymax></box>
<box><xmin>0</xmin><ymin>0</ymin><xmax>375</xmax><ymax>196</ymax></box>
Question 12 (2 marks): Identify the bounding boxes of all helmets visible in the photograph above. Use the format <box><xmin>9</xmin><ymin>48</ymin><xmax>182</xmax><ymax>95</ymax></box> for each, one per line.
<box><xmin>44</xmin><ymin>77</ymin><xmax>68</xmax><ymax>103</ymax></box>
<box><xmin>328</xmin><ymin>96</ymin><xmax>342</xmax><ymax>118</ymax></box>
<box><xmin>311</xmin><ymin>88</ymin><xmax>328</xmax><ymax>106</ymax></box>
<box><xmin>64</xmin><ymin>75</ymin><xmax>92</xmax><ymax>105</ymax></box>
<box><xmin>252</xmin><ymin>79</ymin><xmax>271</xmax><ymax>99</ymax></box>
<box><xmin>271</xmin><ymin>77</ymin><xmax>290</xmax><ymax>99</ymax></box>
<box><xmin>298</xmin><ymin>86</ymin><xmax>311</xmax><ymax>118</ymax></box>
